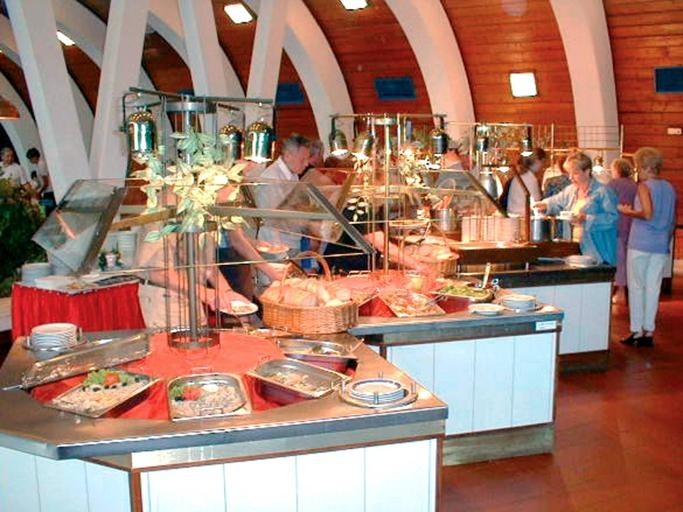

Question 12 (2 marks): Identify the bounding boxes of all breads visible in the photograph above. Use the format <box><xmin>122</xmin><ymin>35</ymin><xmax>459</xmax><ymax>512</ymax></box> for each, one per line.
<box><xmin>262</xmin><ymin>278</ymin><xmax>353</xmax><ymax>307</ymax></box>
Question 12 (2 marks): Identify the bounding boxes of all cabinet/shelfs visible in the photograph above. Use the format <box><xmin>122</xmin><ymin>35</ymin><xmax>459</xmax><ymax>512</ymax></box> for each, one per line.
<box><xmin>472</xmin><ymin>123</ymin><xmax>626</xmax><ymax>170</ymax></box>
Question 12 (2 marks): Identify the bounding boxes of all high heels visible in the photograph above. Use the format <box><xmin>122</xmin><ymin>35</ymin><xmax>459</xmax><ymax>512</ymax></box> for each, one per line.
<box><xmin>643</xmin><ymin>336</ymin><xmax>653</xmax><ymax>348</ymax></box>
<box><xmin>620</xmin><ymin>334</ymin><xmax>642</xmax><ymax>348</ymax></box>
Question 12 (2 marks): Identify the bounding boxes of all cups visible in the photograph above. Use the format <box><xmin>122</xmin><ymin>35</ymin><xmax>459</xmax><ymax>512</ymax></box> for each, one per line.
<box><xmin>105</xmin><ymin>253</ymin><xmax>117</xmax><ymax>269</ymax></box>
<box><xmin>559</xmin><ymin>210</ymin><xmax>574</xmax><ymax>217</ymax></box>
<box><xmin>458</xmin><ymin>213</ymin><xmax>521</xmax><ymax>242</ymax></box>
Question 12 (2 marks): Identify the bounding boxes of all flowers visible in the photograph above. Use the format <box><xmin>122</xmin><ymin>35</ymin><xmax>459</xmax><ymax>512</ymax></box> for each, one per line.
<box><xmin>129</xmin><ymin>123</ymin><xmax>268</xmax><ymax>252</ymax></box>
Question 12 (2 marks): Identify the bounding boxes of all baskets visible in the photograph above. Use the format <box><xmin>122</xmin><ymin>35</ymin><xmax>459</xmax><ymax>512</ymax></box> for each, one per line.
<box><xmin>404</xmin><ymin>223</ymin><xmax>460</xmax><ymax>277</ymax></box>
<box><xmin>260</xmin><ymin>251</ymin><xmax>359</xmax><ymax>335</ymax></box>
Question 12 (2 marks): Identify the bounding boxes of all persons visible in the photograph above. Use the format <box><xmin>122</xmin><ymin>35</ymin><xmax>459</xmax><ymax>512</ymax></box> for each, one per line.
<box><xmin>499</xmin><ymin>148</ymin><xmax>677</xmax><ymax>348</ymax></box>
<box><xmin>133</xmin><ymin>133</ymin><xmax>463</xmax><ymax>328</ymax></box>
<box><xmin>0</xmin><ymin>147</ymin><xmax>57</xmax><ymax>218</ymax></box>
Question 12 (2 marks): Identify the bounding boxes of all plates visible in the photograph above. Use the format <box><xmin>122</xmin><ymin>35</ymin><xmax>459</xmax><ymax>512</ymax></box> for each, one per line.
<box><xmin>500</xmin><ymin>292</ymin><xmax>536</xmax><ymax>308</ymax></box>
<box><xmin>569</xmin><ymin>253</ymin><xmax>592</xmax><ymax>265</ymax></box>
<box><xmin>255</xmin><ymin>244</ymin><xmax>290</xmax><ymax>254</ymax></box>
<box><xmin>350</xmin><ymin>379</ymin><xmax>404</xmax><ymax>401</ymax></box>
<box><xmin>103</xmin><ymin>230</ymin><xmax>140</xmax><ymax>254</ymax></box>
<box><xmin>31</xmin><ymin>322</ymin><xmax>78</xmax><ymax>347</ymax></box>
<box><xmin>556</xmin><ymin>216</ymin><xmax>576</xmax><ymax>221</ymax></box>
<box><xmin>466</xmin><ymin>301</ymin><xmax>503</xmax><ymax>316</ymax></box>
<box><xmin>219</xmin><ymin>300</ymin><xmax>258</xmax><ymax>315</ymax></box>
<box><xmin>21</xmin><ymin>262</ymin><xmax>53</xmax><ymax>286</ymax></box>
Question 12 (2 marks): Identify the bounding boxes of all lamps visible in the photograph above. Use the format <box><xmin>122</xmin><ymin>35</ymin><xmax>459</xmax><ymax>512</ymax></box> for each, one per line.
<box><xmin>591</xmin><ymin>150</ymin><xmax>605</xmax><ymax>175</ymax></box>
<box><xmin>0</xmin><ymin>94</ymin><xmax>20</xmax><ymax>121</ymax></box>
<box><xmin>116</xmin><ymin>83</ymin><xmax>278</xmax><ymax>351</ymax></box>
<box><xmin>325</xmin><ymin>110</ymin><xmax>534</xmax><ymax>281</ymax></box>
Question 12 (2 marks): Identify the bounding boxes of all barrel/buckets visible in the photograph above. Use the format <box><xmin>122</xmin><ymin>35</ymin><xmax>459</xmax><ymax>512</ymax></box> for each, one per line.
<box><xmin>530</xmin><ymin>217</ymin><xmax>551</xmax><ymax>242</ymax></box>
<box><xmin>440</xmin><ymin>208</ymin><xmax>458</xmax><ymax>231</ymax></box>
<box><xmin>555</xmin><ymin>217</ymin><xmax>574</xmax><ymax>241</ymax></box>
